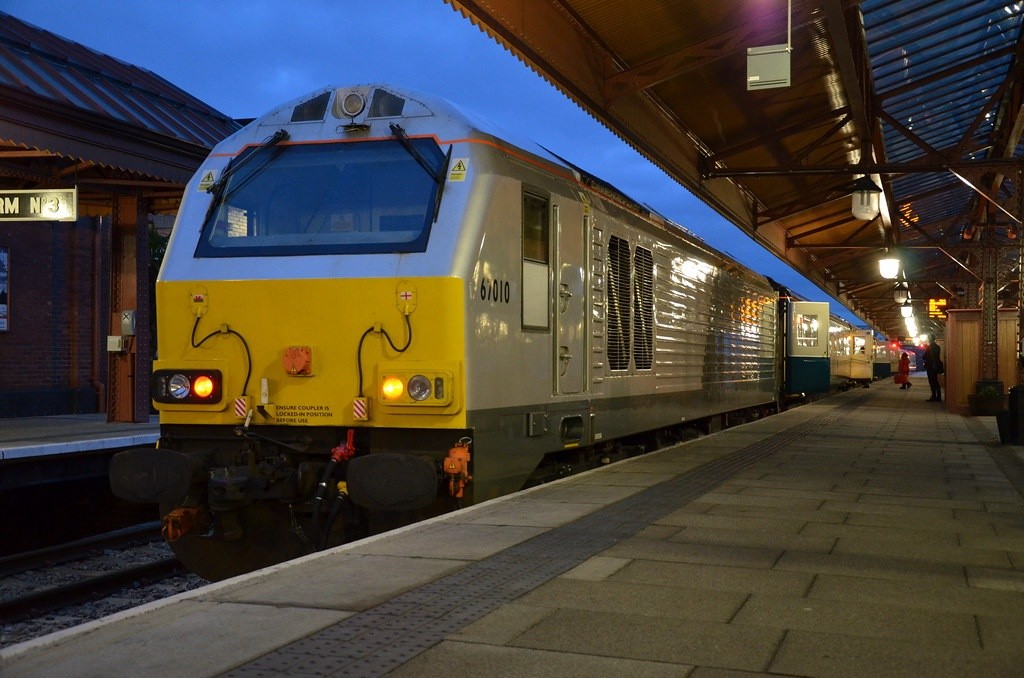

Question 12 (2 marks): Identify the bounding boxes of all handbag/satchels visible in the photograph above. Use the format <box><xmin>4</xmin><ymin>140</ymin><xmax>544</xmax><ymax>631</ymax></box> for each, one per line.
<box><xmin>936</xmin><ymin>361</ymin><xmax>944</xmax><ymax>373</ymax></box>
<box><xmin>895</xmin><ymin>372</ymin><xmax>908</xmax><ymax>383</ymax></box>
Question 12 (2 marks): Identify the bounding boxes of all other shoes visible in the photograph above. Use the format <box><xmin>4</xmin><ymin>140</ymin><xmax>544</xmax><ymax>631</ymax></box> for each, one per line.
<box><xmin>927</xmin><ymin>397</ymin><xmax>937</xmax><ymax>402</ymax></box>
<box><xmin>907</xmin><ymin>383</ymin><xmax>911</xmax><ymax>388</ymax></box>
<box><xmin>900</xmin><ymin>387</ymin><xmax>906</xmax><ymax>389</ymax></box>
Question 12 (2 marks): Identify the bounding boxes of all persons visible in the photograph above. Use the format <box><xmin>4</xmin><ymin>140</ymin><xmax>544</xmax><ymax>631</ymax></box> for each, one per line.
<box><xmin>923</xmin><ymin>334</ymin><xmax>944</xmax><ymax>402</ymax></box>
<box><xmin>861</xmin><ymin>347</ymin><xmax>870</xmax><ymax>388</ymax></box>
<box><xmin>898</xmin><ymin>352</ymin><xmax>912</xmax><ymax>390</ymax></box>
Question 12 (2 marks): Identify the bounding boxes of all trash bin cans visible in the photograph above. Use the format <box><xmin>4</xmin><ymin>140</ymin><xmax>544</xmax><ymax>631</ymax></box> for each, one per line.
<box><xmin>995</xmin><ymin>385</ymin><xmax>1024</xmax><ymax>445</ymax></box>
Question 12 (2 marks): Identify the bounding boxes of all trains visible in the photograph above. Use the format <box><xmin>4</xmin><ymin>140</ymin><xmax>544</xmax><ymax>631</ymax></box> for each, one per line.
<box><xmin>149</xmin><ymin>81</ymin><xmax>916</xmax><ymax>587</ymax></box>
